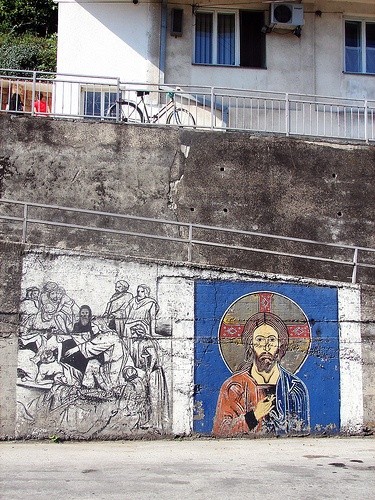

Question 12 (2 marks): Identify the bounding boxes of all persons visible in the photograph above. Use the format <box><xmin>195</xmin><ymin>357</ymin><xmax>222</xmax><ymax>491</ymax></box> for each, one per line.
<box><xmin>34</xmin><ymin>92</ymin><xmax>51</xmax><ymax>117</ymax></box>
<box><xmin>8</xmin><ymin>84</ymin><xmax>25</xmax><ymax>115</ymax></box>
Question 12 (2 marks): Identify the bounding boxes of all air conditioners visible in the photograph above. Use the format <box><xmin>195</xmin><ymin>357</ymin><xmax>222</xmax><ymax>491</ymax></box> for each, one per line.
<box><xmin>268</xmin><ymin>2</ymin><xmax>306</xmax><ymax>30</ymax></box>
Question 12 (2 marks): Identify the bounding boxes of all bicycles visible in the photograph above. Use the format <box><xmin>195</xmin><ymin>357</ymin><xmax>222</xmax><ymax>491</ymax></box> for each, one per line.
<box><xmin>105</xmin><ymin>88</ymin><xmax>196</xmax><ymax>130</ymax></box>
